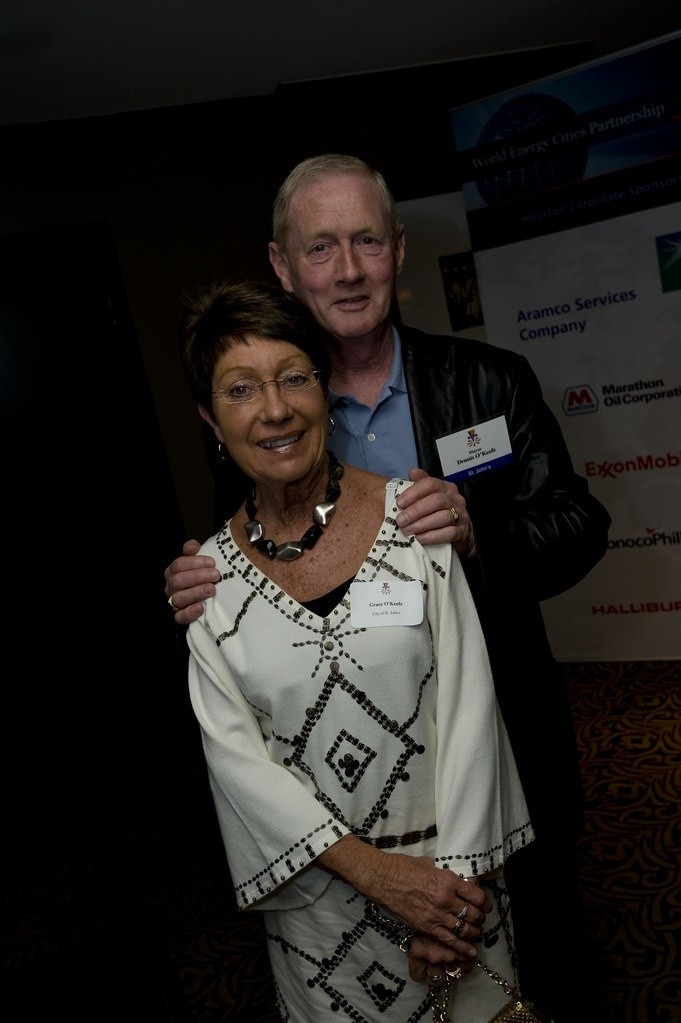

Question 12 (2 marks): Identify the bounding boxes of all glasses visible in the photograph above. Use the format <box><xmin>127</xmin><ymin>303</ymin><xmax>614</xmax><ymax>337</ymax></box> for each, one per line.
<box><xmin>211</xmin><ymin>368</ymin><xmax>320</xmax><ymax>405</ymax></box>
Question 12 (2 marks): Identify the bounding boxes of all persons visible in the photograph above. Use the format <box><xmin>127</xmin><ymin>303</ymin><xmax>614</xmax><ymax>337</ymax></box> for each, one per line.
<box><xmin>164</xmin><ymin>155</ymin><xmax>622</xmax><ymax>1023</ymax></box>
<box><xmin>183</xmin><ymin>282</ymin><xmax>552</xmax><ymax>1023</ymax></box>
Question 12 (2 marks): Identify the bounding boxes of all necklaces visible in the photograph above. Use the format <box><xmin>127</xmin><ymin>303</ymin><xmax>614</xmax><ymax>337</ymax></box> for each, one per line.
<box><xmin>244</xmin><ymin>449</ymin><xmax>343</xmax><ymax>561</ymax></box>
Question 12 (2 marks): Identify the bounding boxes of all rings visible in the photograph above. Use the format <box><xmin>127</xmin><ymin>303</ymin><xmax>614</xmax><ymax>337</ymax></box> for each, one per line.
<box><xmin>449</xmin><ymin>506</ymin><xmax>459</xmax><ymax>526</ymax></box>
<box><xmin>426</xmin><ymin>969</ymin><xmax>445</xmax><ymax>983</ymax></box>
<box><xmin>456</xmin><ymin>899</ymin><xmax>469</xmax><ymax>919</ymax></box>
<box><xmin>448</xmin><ymin>919</ymin><xmax>465</xmax><ymax>936</ymax></box>
<box><xmin>443</xmin><ymin>967</ymin><xmax>462</xmax><ymax>979</ymax></box>
<box><xmin>167</xmin><ymin>595</ymin><xmax>180</xmax><ymax>613</ymax></box>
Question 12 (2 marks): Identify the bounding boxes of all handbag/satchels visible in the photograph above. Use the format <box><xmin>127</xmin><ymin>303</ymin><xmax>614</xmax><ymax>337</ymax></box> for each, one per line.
<box><xmin>486</xmin><ymin>997</ymin><xmax>556</xmax><ymax>1023</ymax></box>
